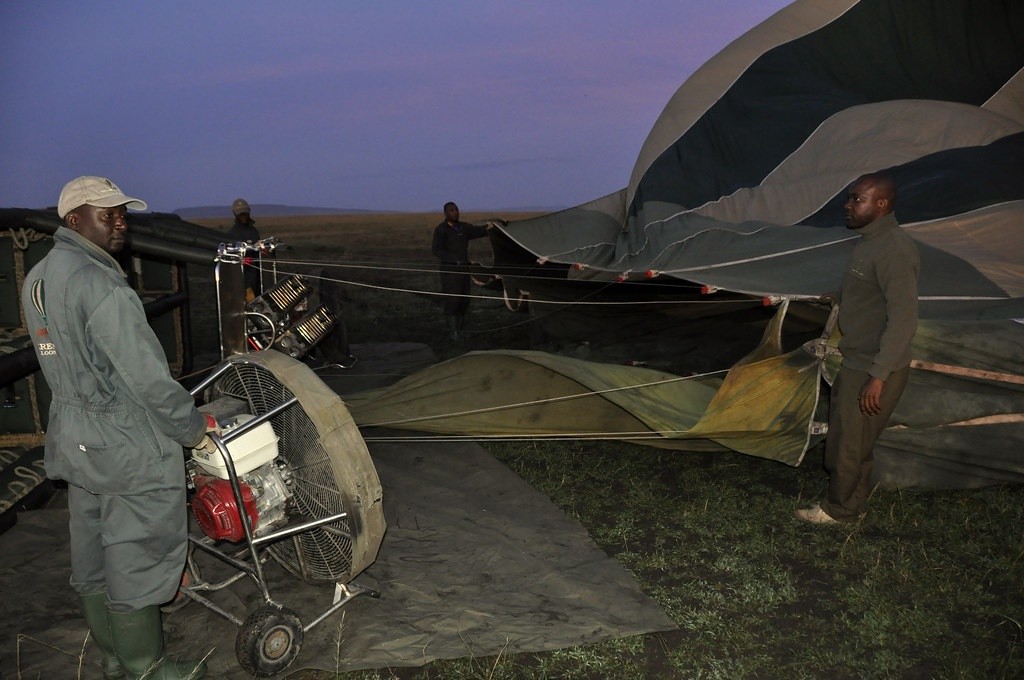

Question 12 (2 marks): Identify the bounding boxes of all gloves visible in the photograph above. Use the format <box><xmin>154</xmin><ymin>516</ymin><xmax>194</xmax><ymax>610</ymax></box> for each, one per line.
<box><xmin>192</xmin><ymin>416</ymin><xmax>222</xmax><ymax>453</ymax></box>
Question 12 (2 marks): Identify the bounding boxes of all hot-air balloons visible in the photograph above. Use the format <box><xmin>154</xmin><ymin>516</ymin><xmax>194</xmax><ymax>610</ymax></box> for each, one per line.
<box><xmin>0</xmin><ymin>0</ymin><xmax>1024</xmax><ymax>534</ymax></box>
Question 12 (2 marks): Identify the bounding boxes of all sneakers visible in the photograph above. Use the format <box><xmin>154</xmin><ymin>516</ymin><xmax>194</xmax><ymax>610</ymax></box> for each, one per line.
<box><xmin>793</xmin><ymin>504</ymin><xmax>867</xmax><ymax>526</ymax></box>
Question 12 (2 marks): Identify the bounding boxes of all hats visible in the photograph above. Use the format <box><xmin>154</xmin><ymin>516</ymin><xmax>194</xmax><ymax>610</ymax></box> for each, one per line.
<box><xmin>232</xmin><ymin>199</ymin><xmax>251</xmax><ymax>216</ymax></box>
<box><xmin>57</xmin><ymin>176</ymin><xmax>147</xmax><ymax>220</ymax></box>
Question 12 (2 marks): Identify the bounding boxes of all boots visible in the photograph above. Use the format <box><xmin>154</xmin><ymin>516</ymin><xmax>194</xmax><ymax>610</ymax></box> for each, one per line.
<box><xmin>447</xmin><ymin>314</ymin><xmax>465</xmax><ymax>335</ymax></box>
<box><xmin>77</xmin><ymin>589</ymin><xmax>128</xmax><ymax>680</ymax></box>
<box><xmin>104</xmin><ymin>603</ymin><xmax>208</xmax><ymax>680</ymax></box>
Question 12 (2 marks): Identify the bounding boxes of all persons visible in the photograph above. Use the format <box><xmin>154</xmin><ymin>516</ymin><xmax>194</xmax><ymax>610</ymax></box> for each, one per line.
<box><xmin>792</xmin><ymin>173</ymin><xmax>922</xmax><ymax>526</ymax></box>
<box><xmin>21</xmin><ymin>175</ymin><xmax>222</xmax><ymax>680</ymax></box>
<box><xmin>431</xmin><ymin>202</ymin><xmax>510</xmax><ymax>342</ymax></box>
<box><xmin>227</xmin><ymin>198</ymin><xmax>268</xmax><ymax>296</ymax></box>
